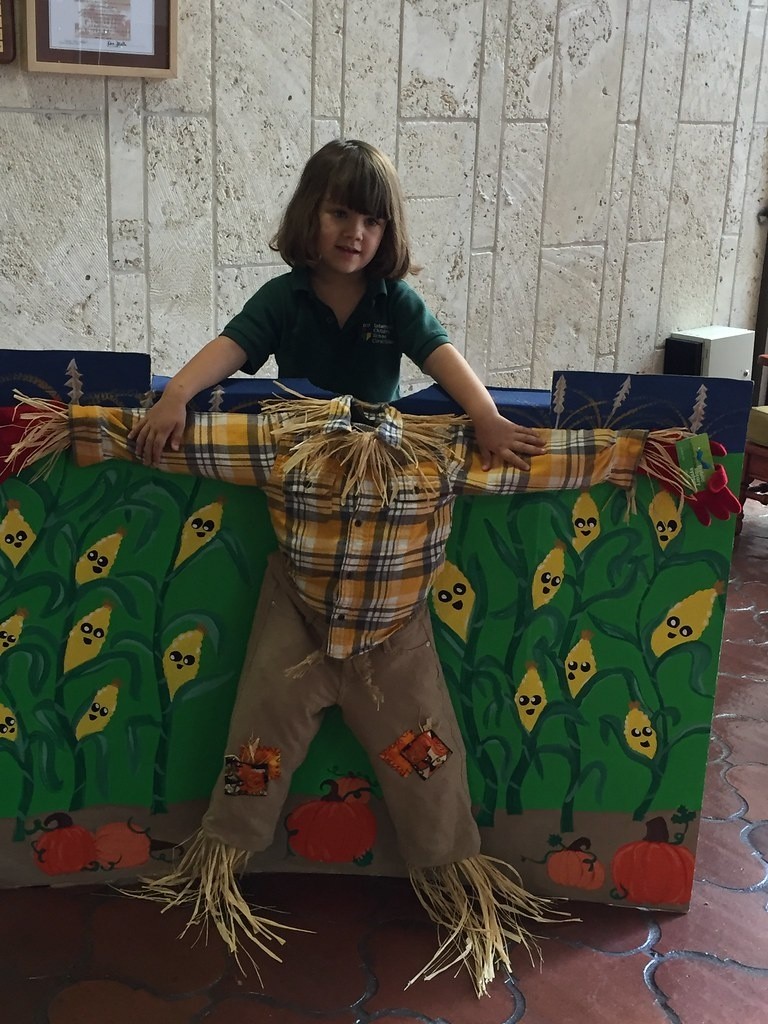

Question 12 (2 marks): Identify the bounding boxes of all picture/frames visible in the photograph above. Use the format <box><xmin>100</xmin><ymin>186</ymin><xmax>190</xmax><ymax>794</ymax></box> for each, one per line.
<box><xmin>0</xmin><ymin>0</ymin><xmax>16</xmax><ymax>66</ymax></box>
<box><xmin>27</xmin><ymin>1</ymin><xmax>176</xmax><ymax>82</ymax></box>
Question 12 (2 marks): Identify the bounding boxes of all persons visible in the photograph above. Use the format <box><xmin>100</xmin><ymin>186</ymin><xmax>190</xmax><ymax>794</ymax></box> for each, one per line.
<box><xmin>128</xmin><ymin>138</ymin><xmax>549</xmax><ymax>474</ymax></box>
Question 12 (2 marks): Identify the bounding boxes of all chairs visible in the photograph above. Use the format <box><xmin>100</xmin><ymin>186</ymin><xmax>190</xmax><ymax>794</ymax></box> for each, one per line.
<box><xmin>736</xmin><ymin>441</ymin><xmax>768</xmax><ymax>535</ymax></box>
